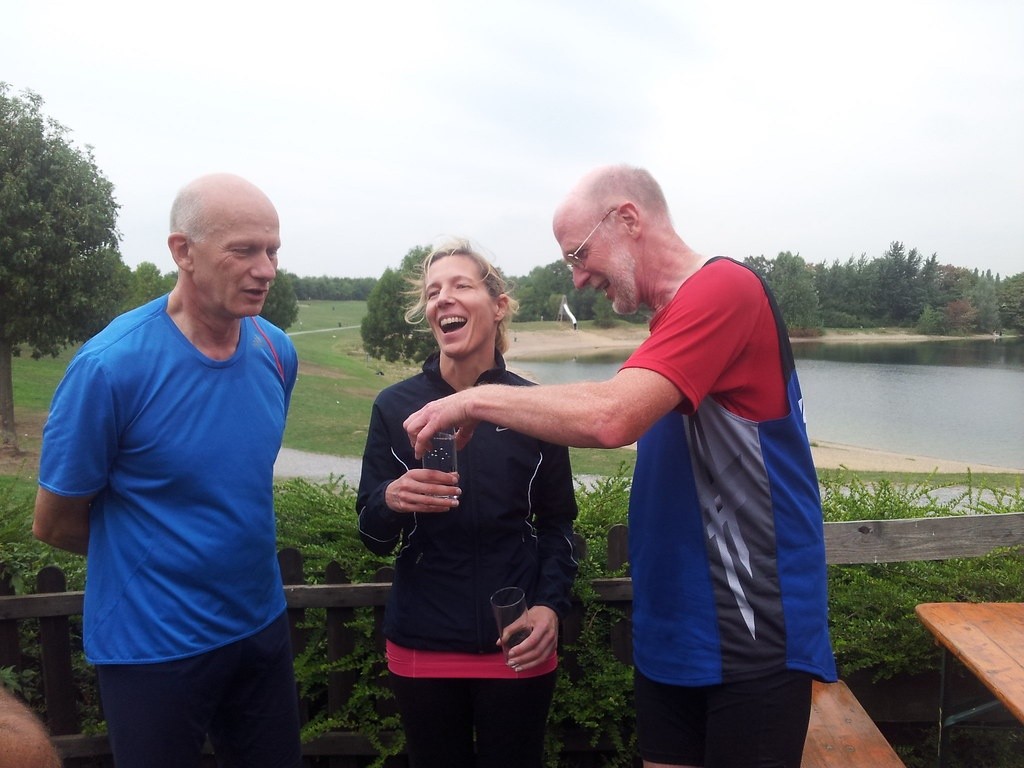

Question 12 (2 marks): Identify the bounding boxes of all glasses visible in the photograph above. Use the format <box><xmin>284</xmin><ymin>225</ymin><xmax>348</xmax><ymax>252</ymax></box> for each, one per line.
<box><xmin>566</xmin><ymin>207</ymin><xmax>617</xmax><ymax>273</ymax></box>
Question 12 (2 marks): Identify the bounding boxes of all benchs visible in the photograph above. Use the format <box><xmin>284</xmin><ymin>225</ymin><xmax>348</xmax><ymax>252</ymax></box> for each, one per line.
<box><xmin>801</xmin><ymin>679</ymin><xmax>907</xmax><ymax>768</ymax></box>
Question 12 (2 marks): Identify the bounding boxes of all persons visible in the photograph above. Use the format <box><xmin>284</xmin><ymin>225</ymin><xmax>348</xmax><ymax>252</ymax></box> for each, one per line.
<box><xmin>32</xmin><ymin>173</ymin><xmax>298</xmax><ymax>768</ymax></box>
<box><xmin>402</xmin><ymin>161</ymin><xmax>838</xmax><ymax>768</ymax></box>
<box><xmin>0</xmin><ymin>688</ymin><xmax>59</xmax><ymax>768</ymax></box>
<box><xmin>354</xmin><ymin>239</ymin><xmax>580</xmax><ymax>768</ymax></box>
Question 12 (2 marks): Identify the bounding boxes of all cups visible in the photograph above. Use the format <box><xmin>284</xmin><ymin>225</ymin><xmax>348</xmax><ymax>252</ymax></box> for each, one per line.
<box><xmin>422</xmin><ymin>431</ymin><xmax>460</xmax><ymax>500</ymax></box>
<box><xmin>490</xmin><ymin>587</ymin><xmax>533</xmax><ymax>666</ymax></box>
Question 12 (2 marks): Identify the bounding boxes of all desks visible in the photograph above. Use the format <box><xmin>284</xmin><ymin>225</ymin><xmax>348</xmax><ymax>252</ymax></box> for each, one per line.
<box><xmin>915</xmin><ymin>601</ymin><xmax>1024</xmax><ymax>768</ymax></box>
